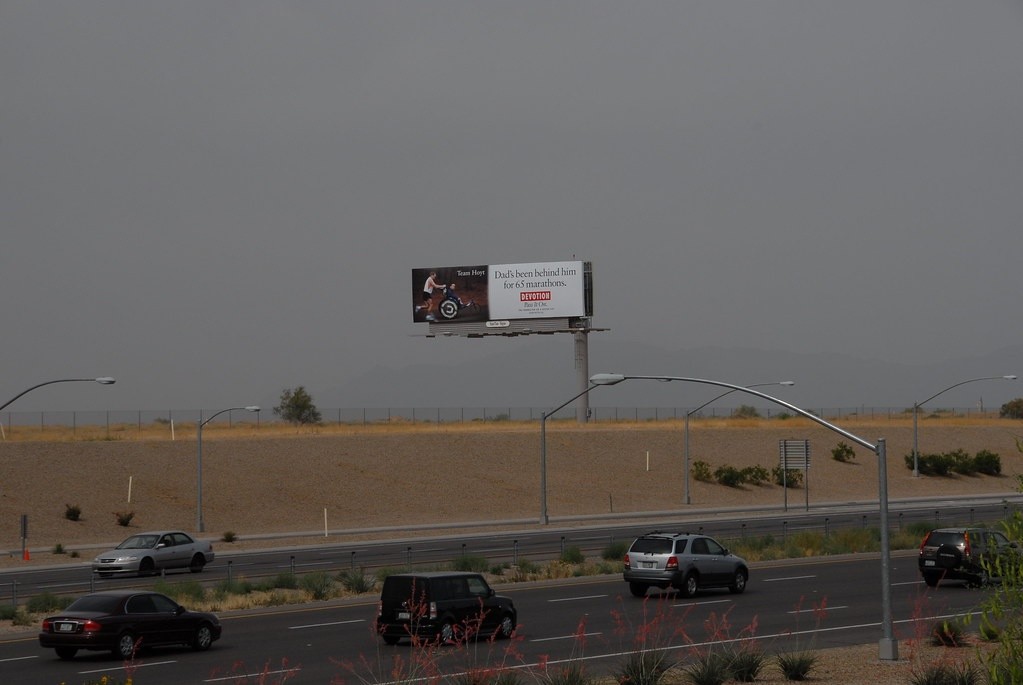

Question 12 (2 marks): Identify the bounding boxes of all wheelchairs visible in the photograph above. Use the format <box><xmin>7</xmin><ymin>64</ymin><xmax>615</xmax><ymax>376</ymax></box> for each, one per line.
<box><xmin>438</xmin><ymin>284</ymin><xmax>481</xmax><ymax>320</ymax></box>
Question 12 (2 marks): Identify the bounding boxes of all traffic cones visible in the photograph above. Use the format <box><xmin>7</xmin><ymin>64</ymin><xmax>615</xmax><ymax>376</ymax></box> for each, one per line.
<box><xmin>24</xmin><ymin>548</ymin><xmax>30</xmax><ymax>561</ymax></box>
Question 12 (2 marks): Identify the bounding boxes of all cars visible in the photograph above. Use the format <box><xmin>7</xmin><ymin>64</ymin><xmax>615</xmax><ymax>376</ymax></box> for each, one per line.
<box><xmin>92</xmin><ymin>530</ymin><xmax>215</xmax><ymax>579</ymax></box>
<box><xmin>38</xmin><ymin>590</ymin><xmax>223</xmax><ymax>660</ymax></box>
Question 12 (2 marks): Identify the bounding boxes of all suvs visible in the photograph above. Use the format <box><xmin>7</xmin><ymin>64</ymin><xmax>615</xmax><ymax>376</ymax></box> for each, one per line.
<box><xmin>376</xmin><ymin>571</ymin><xmax>516</xmax><ymax>646</ymax></box>
<box><xmin>624</xmin><ymin>531</ymin><xmax>750</xmax><ymax>599</ymax></box>
<box><xmin>919</xmin><ymin>527</ymin><xmax>1023</xmax><ymax>588</ymax></box>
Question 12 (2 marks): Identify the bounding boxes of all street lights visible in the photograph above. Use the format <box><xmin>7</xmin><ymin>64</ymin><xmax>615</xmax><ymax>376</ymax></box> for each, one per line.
<box><xmin>683</xmin><ymin>381</ymin><xmax>795</xmax><ymax>505</ymax></box>
<box><xmin>196</xmin><ymin>406</ymin><xmax>260</xmax><ymax>532</ymax></box>
<box><xmin>913</xmin><ymin>375</ymin><xmax>1017</xmax><ymax>477</ymax></box>
<box><xmin>589</xmin><ymin>370</ymin><xmax>897</xmax><ymax>661</ymax></box>
<box><xmin>541</xmin><ymin>373</ymin><xmax>671</xmax><ymax>526</ymax></box>
